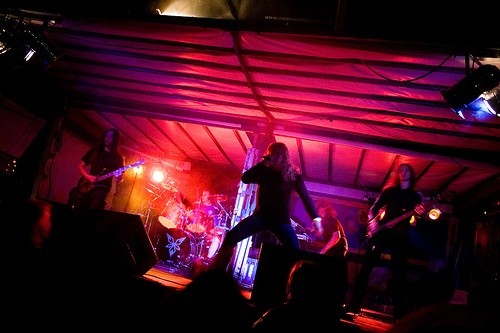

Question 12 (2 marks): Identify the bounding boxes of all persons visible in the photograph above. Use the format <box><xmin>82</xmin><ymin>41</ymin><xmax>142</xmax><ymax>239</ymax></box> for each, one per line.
<box><xmin>210</xmin><ymin>142</ymin><xmax>323</xmax><ymax>272</ymax></box>
<box><xmin>16</xmin><ymin>112</ymin><xmax>64</xmax><ymax>200</ymax></box>
<box><xmin>356</xmin><ymin>164</ymin><xmax>428</xmax><ymax>293</ymax></box>
<box><xmin>251</xmin><ymin>261</ymin><xmax>360</xmax><ymax>333</ymax></box>
<box><xmin>78</xmin><ymin>128</ymin><xmax>124</xmax><ymax>210</ymax></box>
<box><xmin>162</xmin><ymin>270</ymin><xmax>242</xmax><ymax>333</ymax></box>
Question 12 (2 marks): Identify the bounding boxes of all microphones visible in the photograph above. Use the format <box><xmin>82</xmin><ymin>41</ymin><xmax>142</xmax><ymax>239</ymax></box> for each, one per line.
<box><xmin>262</xmin><ymin>155</ymin><xmax>270</xmax><ymax>161</ymax></box>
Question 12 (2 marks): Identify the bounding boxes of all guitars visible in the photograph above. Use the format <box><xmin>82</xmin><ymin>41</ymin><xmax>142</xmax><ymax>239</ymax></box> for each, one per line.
<box><xmin>366</xmin><ymin>188</ymin><xmax>456</xmax><ymax>246</ymax></box>
<box><xmin>74</xmin><ymin>159</ymin><xmax>145</xmax><ymax>194</ymax></box>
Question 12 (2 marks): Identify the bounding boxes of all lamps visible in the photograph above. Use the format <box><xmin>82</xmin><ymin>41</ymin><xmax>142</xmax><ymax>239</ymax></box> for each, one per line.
<box><xmin>438</xmin><ymin>49</ymin><xmax>500</xmax><ymax>112</ymax></box>
<box><xmin>0</xmin><ymin>5</ymin><xmax>65</xmax><ymax>69</ymax></box>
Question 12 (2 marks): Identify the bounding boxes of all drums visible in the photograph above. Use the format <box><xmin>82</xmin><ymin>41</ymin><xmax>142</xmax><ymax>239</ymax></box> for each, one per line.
<box><xmin>157</xmin><ymin>228</ymin><xmax>199</xmax><ymax>268</ymax></box>
<box><xmin>154</xmin><ymin>216</ymin><xmax>177</xmax><ymax>245</ymax></box>
<box><xmin>185</xmin><ymin>224</ymin><xmax>206</xmax><ymax>256</ymax></box>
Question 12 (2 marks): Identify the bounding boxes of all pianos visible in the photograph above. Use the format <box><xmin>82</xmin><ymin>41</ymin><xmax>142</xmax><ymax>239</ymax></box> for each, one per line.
<box><xmin>248</xmin><ymin>218</ymin><xmax>322</xmax><ymax>308</ymax></box>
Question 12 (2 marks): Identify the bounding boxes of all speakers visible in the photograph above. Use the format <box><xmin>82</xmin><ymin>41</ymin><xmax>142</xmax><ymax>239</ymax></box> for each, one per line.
<box><xmin>64</xmin><ymin>205</ymin><xmax>158</xmax><ymax>280</ymax></box>
<box><xmin>250</xmin><ymin>239</ymin><xmax>348</xmax><ymax>315</ymax></box>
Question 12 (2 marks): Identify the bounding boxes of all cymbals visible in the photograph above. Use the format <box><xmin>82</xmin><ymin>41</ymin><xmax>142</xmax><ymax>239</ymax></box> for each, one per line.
<box><xmin>208</xmin><ymin>194</ymin><xmax>228</xmax><ymax>202</ymax></box>
<box><xmin>160</xmin><ymin>181</ymin><xmax>178</xmax><ymax>194</ymax></box>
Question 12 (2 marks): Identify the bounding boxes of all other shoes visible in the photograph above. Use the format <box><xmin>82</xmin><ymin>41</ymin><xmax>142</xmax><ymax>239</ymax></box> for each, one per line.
<box><xmin>337</xmin><ymin>303</ymin><xmax>361</xmax><ymax>314</ymax></box>
